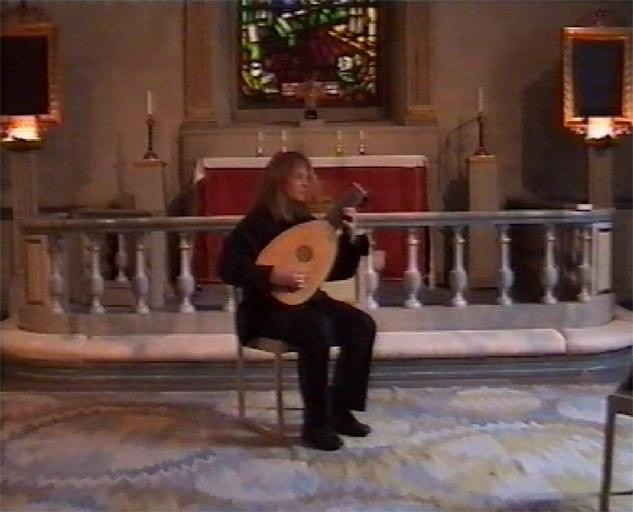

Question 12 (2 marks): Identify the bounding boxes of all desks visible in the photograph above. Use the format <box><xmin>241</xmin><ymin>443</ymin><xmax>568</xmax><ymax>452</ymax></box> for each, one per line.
<box><xmin>193</xmin><ymin>155</ymin><xmax>427</xmax><ymax>284</ymax></box>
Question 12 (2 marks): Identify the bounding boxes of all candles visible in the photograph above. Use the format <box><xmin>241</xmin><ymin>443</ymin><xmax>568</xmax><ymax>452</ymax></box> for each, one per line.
<box><xmin>478</xmin><ymin>86</ymin><xmax>484</xmax><ymax>112</ymax></box>
<box><xmin>146</xmin><ymin>90</ymin><xmax>153</xmax><ymax>116</ymax></box>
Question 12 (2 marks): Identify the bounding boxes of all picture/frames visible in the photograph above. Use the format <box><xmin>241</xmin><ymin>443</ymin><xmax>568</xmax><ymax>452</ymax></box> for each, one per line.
<box><xmin>0</xmin><ymin>18</ymin><xmax>62</xmax><ymax>128</ymax></box>
<box><xmin>563</xmin><ymin>27</ymin><xmax>633</xmax><ymax>137</ymax></box>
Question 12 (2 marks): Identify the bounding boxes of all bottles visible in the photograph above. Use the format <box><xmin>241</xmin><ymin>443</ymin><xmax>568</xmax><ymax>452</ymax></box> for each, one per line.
<box><xmin>279</xmin><ymin>129</ymin><xmax>288</xmax><ymax>153</ymax></box>
<box><xmin>334</xmin><ymin>129</ymin><xmax>344</xmax><ymax>158</ymax></box>
<box><xmin>358</xmin><ymin>129</ymin><xmax>369</xmax><ymax>155</ymax></box>
<box><xmin>256</xmin><ymin>130</ymin><xmax>265</xmax><ymax>157</ymax></box>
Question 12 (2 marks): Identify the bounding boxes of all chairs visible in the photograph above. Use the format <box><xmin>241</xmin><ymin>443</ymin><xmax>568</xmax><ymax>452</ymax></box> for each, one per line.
<box><xmin>234</xmin><ymin>284</ymin><xmax>300</xmax><ymax>427</ymax></box>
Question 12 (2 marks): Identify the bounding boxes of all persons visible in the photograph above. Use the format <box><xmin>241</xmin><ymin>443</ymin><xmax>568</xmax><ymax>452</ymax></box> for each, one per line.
<box><xmin>214</xmin><ymin>150</ymin><xmax>377</xmax><ymax>453</ymax></box>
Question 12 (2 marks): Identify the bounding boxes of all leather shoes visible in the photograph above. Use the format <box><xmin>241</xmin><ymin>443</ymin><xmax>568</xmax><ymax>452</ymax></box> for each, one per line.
<box><xmin>302</xmin><ymin>424</ymin><xmax>342</xmax><ymax>450</ymax></box>
<box><xmin>331</xmin><ymin>410</ymin><xmax>368</xmax><ymax>435</ymax></box>
